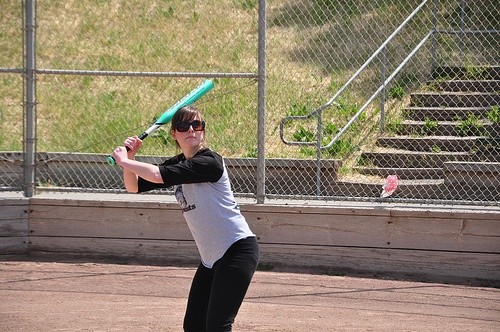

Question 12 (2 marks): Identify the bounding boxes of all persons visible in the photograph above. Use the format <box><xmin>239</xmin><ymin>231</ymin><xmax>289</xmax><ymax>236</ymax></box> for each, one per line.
<box><xmin>113</xmin><ymin>106</ymin><xmax>260</xmax><ymax>331</ymax></box>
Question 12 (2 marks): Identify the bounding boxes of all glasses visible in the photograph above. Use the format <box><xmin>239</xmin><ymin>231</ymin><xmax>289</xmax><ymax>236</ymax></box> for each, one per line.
<box><xmin>174</xmin><ymin>120</ymin><xmax>205</xmax><ymax>132</ymax></box>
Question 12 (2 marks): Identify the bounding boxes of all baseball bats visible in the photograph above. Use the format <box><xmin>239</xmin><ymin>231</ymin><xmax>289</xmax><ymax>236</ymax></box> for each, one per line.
<box><xmin>107</xmin><ymin>79</ymin><xmax>213</xmax><ymax>166</ymax></box>
